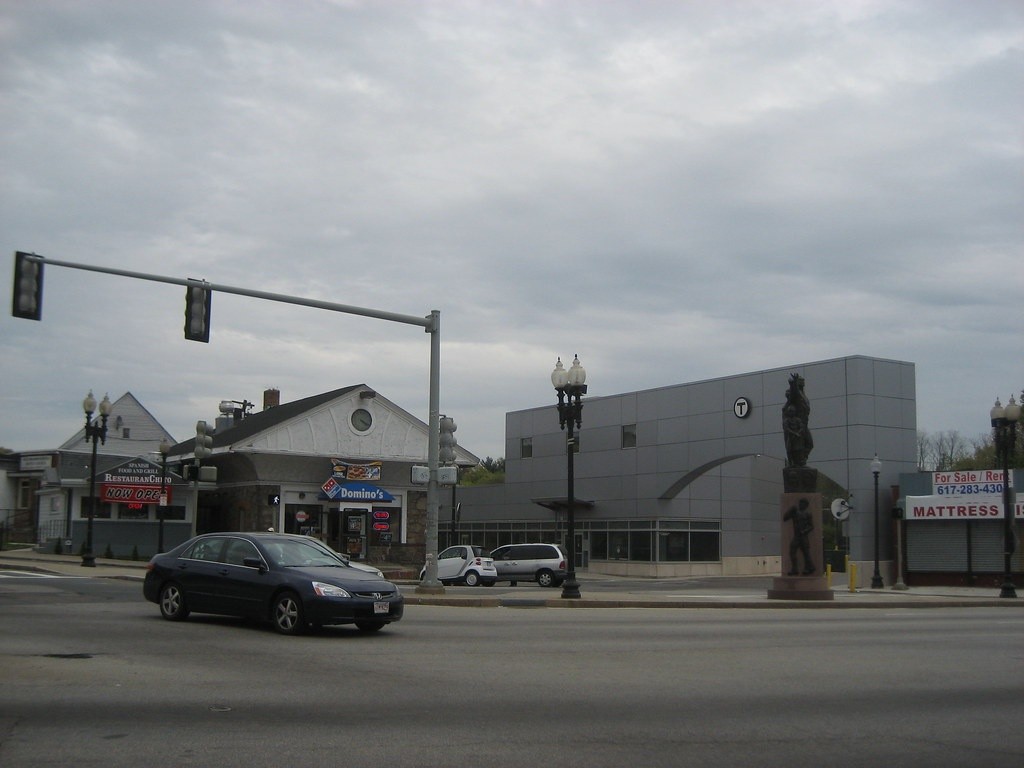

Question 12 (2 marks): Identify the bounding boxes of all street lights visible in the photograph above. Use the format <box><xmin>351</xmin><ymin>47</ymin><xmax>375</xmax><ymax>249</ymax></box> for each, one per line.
<box><xmin>990</xmin><ymin>394</ymin><xmax>1021</xmax><ymax>597</ymax></box>
<box><xmin>869</xmin><ymin>453</ymin><xmax>884</xmax><ymax>589</ymax></box>
<box><xmin>552</xmin><ymin>352</ymin><xmax>588</xmax><ymax>600</ymax></box>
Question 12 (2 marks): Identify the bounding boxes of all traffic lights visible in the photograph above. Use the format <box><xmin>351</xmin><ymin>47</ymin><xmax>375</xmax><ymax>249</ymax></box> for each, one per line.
<box><xmin>187</xmin><ymin>465</ymin><xmax>199</xmax><ymax>481</ymax></box>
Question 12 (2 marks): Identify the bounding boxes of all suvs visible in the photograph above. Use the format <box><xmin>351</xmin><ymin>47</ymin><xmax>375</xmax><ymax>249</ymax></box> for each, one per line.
<box><xmin>490</xmin><ymin>543</ymin><xmax>569</xmax><ymax>588</ymax></box>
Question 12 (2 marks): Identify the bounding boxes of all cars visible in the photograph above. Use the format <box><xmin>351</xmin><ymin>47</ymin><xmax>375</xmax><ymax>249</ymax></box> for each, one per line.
<box><xmin>144</xmin><ymin>532</ymin><xmax>404</xmax><ymax>636</ymax></box>
<box><xmin>294</xmin><ymin>542</ymin><xmax>384</xmax><ymax>579</ymax></box>
<box><xmin>419</xmin><ymin>544</ymin><xmax>497</xmax><ymax>587</ymax></box>
<box><xmin>82</xmin><ymin>389</ymin><xmax>112</xmax><ymax>567</ymax></box>
<box><xmin>157</xmin><ymin>437</ymin><xmax>172</xmax><ymax>553</ymax></box>
